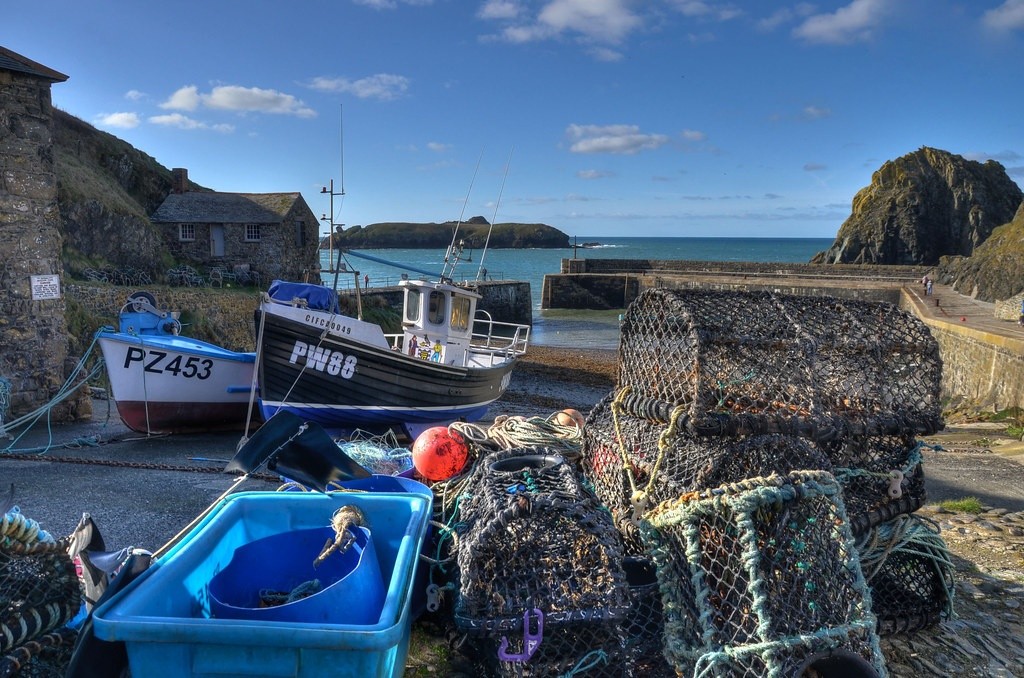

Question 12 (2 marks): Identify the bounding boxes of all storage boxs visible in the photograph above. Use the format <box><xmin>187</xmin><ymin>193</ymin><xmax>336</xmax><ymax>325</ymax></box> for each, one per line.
<box><xmin>91</xmin><ymin>492</ymin><xmax>432</xmax><ymax>678</ymax></box>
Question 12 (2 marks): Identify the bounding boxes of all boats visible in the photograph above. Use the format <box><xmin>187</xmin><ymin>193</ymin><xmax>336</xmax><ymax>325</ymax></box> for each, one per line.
<box><xmin>253</xmin><ymin>145</ymin><xmax>530</xmax><ymax>442</ymax></box>
<box><xmin>94</xmin><ymin>291</ymin><xmax>256</xmax><ymax>434</ymax></box>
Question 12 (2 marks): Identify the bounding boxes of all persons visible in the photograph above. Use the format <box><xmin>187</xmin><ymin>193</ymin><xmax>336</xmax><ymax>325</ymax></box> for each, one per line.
<box><xmin>923</xmin><ymin>276</ymin><xmax>933</xmax><ymax>295</ymax></box>
<box><xmin>364</xmin><ymin>275</ymin><xmax>369</xmax><ymax>288</ymax></box>
<box><xmin>482</xmin><ymin>268</ymin><xmax>487</xmax><ymax>280</ymax></box>
